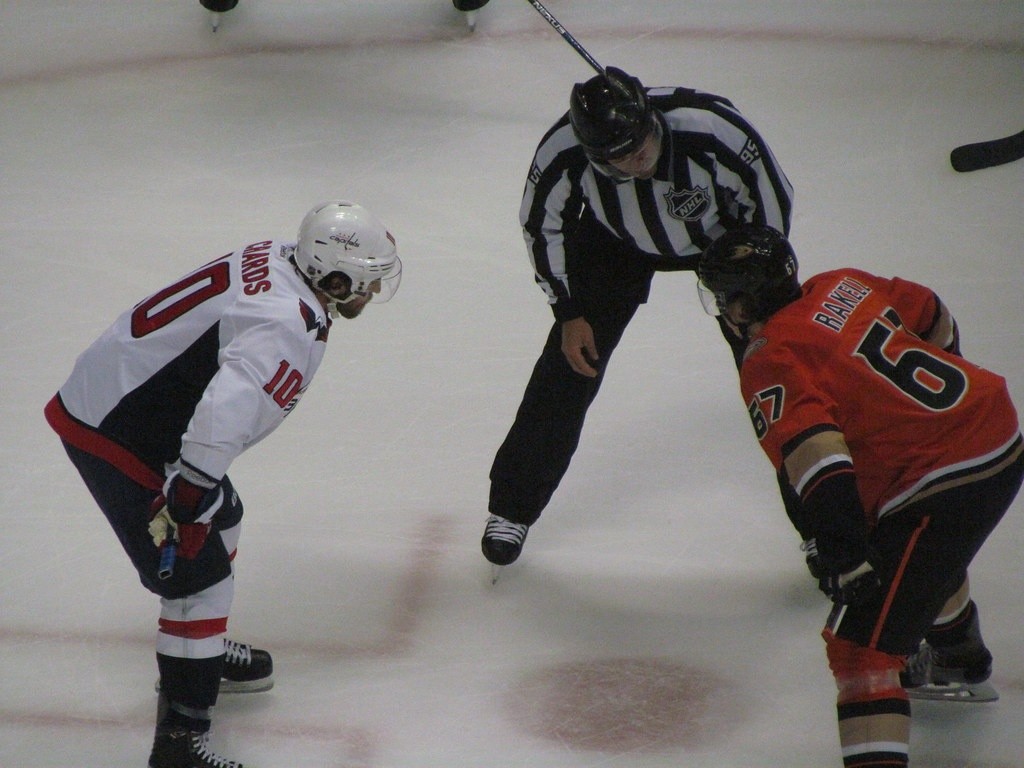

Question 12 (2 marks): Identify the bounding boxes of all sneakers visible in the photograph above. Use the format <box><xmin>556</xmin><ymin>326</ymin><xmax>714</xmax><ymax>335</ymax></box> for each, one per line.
<box><xmin>899</xmin><ymin>597</ymin><xmax>1000</xmax><ymax>702</ymax></box>
<box><xmin>155</xmin><ymin>638</ymin><xmax>275</xmax><ymax>693</ymax></box>
<box><xmin>482</xmin><ymin>513</ymin><xmax>529</xmax><ymax>584</ymax></box>
<box><xmin>147</xmin><ymin>726</ymin><xmax>243</xmax><ymax>768</ymax></box>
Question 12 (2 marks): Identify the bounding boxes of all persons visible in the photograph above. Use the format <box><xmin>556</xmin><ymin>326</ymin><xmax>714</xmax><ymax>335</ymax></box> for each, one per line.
<box><xmin>480</xmin><ymin>66</ymin><xmax>824</xmax><ymax>580</ymax></box>
<box><xmin>694</xmin><ymin>220</ymin><xmax>1024</xmax><ymax>768</ymax></box>
<box><xmin>42</xmin><ymin>199</ymin><xmax>401</xmax><ymax>768</ymax></box>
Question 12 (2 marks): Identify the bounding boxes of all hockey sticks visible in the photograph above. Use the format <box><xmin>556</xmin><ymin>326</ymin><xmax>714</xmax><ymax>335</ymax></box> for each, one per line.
<box><xmin>524</xmin><ymin>0</ymin><xmax>607</xmax><ymax>76</ymax></box>
<box><xmin>155</xmin><ymin>526</ymin><xmax>178</xmax><ymax>579</ymax></box>
<box><xmin>948</xmin><ymin>126</ymin><xmax>1023</xmax><ymax>175</ymax></box>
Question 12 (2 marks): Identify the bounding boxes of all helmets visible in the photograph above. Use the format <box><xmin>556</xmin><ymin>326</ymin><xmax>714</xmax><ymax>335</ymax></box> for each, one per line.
<box><xmin>569</xmin><ymin>65</ymin><xmax>663</xmax><ymax>166</ymax></box>
<box><xmin>697</xmin><ymin>223</ymin><xmax>799</xmax><ymax>316</ymax></box>
<box><xmin>294</xmin><ymin>199</ymin><xmax>402</xmax><ymax>303</ymax></box>
